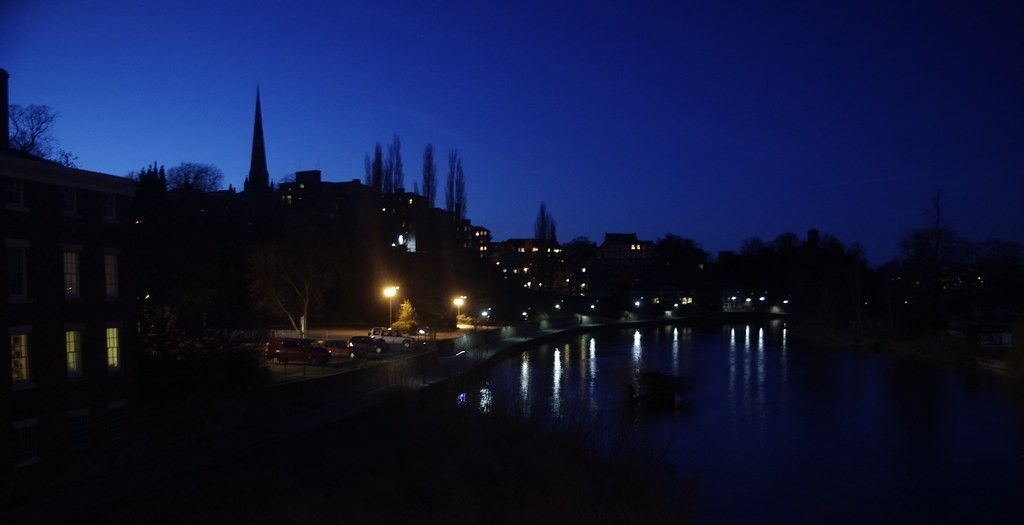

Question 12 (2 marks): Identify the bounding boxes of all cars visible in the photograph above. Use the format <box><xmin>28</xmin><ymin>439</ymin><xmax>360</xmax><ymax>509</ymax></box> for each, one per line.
<box><xmin>350</xmin><ymin>336</ymin><xmax>386</xmax><ymax>354</ymax></box>
<box><xmin>369</xmin><ymin>327</ymin><xmax>415</xmax><ymax>350</ymax></box>
<box><xmin>318</xmin><ymin>340</ymin><xmax>362</xmax><ymax>357</ymax></box>
<box><xmin>269</xmin><ymin>336</ymin><xmax>328</xmax><ymax>364</ymax></box>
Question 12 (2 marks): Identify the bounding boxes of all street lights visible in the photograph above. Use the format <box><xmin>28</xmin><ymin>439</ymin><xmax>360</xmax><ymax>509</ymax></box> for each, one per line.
<box><xmin>385</xmin><ymin>286</ymin><xmax>396</xmax><ymax>331</ymax></box>
<box><xmin>455</xmin><ymin>298</ymin><xmax>462</xmax><ymax>323</ymax></box>
